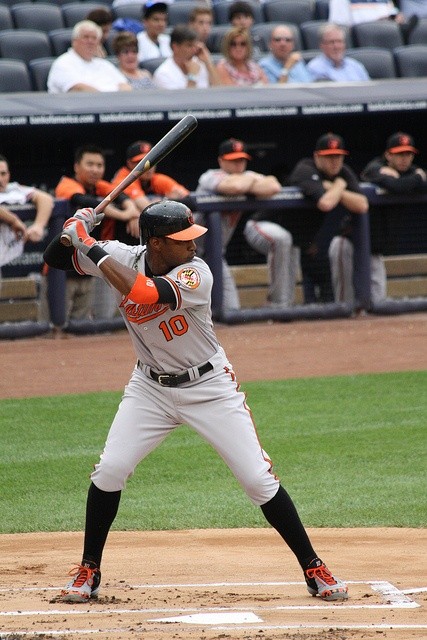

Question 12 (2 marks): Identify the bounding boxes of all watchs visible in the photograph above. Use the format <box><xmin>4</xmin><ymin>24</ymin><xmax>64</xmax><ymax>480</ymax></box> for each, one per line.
<box><xmin>281</xmin><ymin>67</ymin><xmax>292</xmax><ymax>79</ymax></box>
<box><xmin>187</xmin><ymin>75</ymin><xmax>199</xmax><ymax>83</ymax></box>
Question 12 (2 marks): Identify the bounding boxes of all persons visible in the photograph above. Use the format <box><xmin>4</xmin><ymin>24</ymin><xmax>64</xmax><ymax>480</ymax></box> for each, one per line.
<box><xmin>226</xmin><ymin>2</ymin><xmax>266</xmax><ymax>53</ymax></box>
<box><xmin>45</xmin><ymin>19</ymin><xmax>134</xmax><ymax>93</ymax></box>
<box><xmin>194</xmin><ymin>139</ymin><xmax>295</xmax><ymax>311</ymax></box>
<box><xmin>116</xmin><ymin>32</ymin><xmax>154</xmax><ymax>92</ymax></box>
<box><xmin>328</xmin><ymin>0</ymin><xmax>420</xmax><ymax>48</ymax></box>
<box><xmin>136</xmin><ymin>3</ymin><xmax>174</xmax><ymax>64</ymax></box>
<box><xmin>217</xmin><ymin>28</ymin><xmax>270</xmax><ymax>87</ymax></box>
<box><xmin>86</xmin><ymin>10</ymin><xmax>115</xmax><ymax>58</ymax></box>
<box><xmin>154</xmin><ymin>28</ymin><xmax>225</xmax><ymax>90</ymax></box>
<box><xmin>360</xmin><ymin>132</ymin><xmax>427</xmax><ymax>258</ymax></box>
<box><xmin>0</xmin><ymin>160</ymin><xmax>54</xmax><ymax>268</ymax></box>
<box><xmin>60</xmin><ymin>201</ymin><xmax>351</xmax><ymax>604</ymax></box>
<box><xmin>259</xmin><ymin>25</ymin><xmax>316</xmax><ymax>85</ymax></box>
<box><xmin>280</xmin><ymin>134</ymin><xmax>374</xmax><ymax>303</ymax></box>
<box><xmin>187</xmin><ymin>8</ymin><xmax>221</xmax><ymax>56</ymax></box>
<box><xmin>94</xmin><ymin>142</ymin><xmax>196</xmax><ymax>320</ymax></box>
<box><xmin>37</xmin><ymin>150</ymin><xmax>141</xmax><ymax>327</ymax></box>
<box><xmin>306</xmin><ymin>25</ymin><xmax>373</xmax><ymax>85</ymax></box>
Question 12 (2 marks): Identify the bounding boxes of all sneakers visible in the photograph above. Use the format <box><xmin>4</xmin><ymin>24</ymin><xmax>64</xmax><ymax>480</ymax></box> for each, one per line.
<box><xmin>60</xmin><ymin>560</ymin><xmax>102</xmax><ymax>603</ymax></box>
<box><xmin>303</xmin><ymin>558</ymin><xmax>348</xmax><ymax>601</ymax></box>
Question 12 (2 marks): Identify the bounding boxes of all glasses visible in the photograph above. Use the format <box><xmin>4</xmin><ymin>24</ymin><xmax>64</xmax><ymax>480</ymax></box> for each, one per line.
<box><xmin>230</xmin><ymin>41</ymin><xmax>247</xmax><ymax>47</ymax></box>
<box><xmin>272</xmin><ymin>37</ymin><xmax>292</xmax><ymax>43</ymax></box>
<box><xmin>118</xmin><ymin>48</ymin><xmax>137</xmax><ymax>55</ymax></box>
<box><xmin>322</xmin><ymin>40</ymin><xmax>345</xmax><ymax>45</ymax></box>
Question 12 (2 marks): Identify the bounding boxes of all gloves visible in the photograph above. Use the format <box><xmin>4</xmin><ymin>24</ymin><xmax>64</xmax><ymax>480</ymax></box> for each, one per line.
<box><xmin>63</xmin><ymin>208</ymin><xmax>105</xmax><ymax>234</ymax></box>
<box><xmin>61</xmin><ymin>220</ymin><xmax>111</xmax><ymax>268</ymax></box>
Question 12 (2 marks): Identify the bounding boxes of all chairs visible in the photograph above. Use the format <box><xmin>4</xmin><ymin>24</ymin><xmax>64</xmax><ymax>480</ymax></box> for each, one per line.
<box><xmin>140</xmin><ymin>56</ymin><xmax>169</xmax><ymax>71</ymax></box>
<box><xmin>0</xmin><ymin>6</ymin><xmax>13</xmax><ymax>29</ymax></box>
<box><xmin>394</xmin><ymin>43</ymin><xmax>427</xmax><ymax>78</ymax></box>
<box><xmin>29</xmin><ymin>57</ymin><xmax>56</xmax><ymax>93</ymax></box>
<box><xmin>405</xmin><ymin>19</ymin><xmax>427</xmax><ymax>44</ymax></box>
<box><xmin>113</xmin><ymin>3</ymin><xmax>146</xmax><ymax>20</ymax></box>
<box><xmin>13</xmin><ymin>7</ymin><xmax>65</xmax><ymax>29</ymax></box>
<box><xmin>1</xmin><ymin>58</ymin><xmax>29</xmax><ymax>93</ymax></box>
<box><xmin>213</xmin><ymin>0</ymin><xmax>265</xmax><ymax>23</ymax></box>
<box><xmin>265</xmin><ymin>0</ymin><xmax>315</xmax><ymax>22</ymax></box>
<box><xmin>1</xmin><ymin>30</ymin><xmax>51</xmax><ymax>58</ymax></box>
<box><xmin>304</xmin><ymin>22</ymin><xmax>355</xmax><ymax>51</ymax></box>
<box><xmin>254</xmin><ymin>22</ymin><xmax>303</xmax><ymax>51</ymax></box>
<box><xmin>348</xmin><ymin>48</ymin><xmax>395</xmax><ymax>79</ymax></box>
<box><xmin>51</xmin><ymin>29</ymin><xmax>76</xmax><ymax>54</ymax></box>
<box><xmin>354</xmin><ymin>21</ymin><xmax>405</xmax><ymax>47</ymax></box>
<box><xmin>65</xmin><ymin>5</ymin><xmax>112</xmax><ymax>30</ymax></box>
<box><xmin>166</xmin><ymin>2</ymin><xmax>213</xmax><ymax>24</ymax></box>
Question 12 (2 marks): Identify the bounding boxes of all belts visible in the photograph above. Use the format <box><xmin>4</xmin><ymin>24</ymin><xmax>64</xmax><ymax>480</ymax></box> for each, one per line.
<box><xmin>137</xmin><ymin>360</ymin><xmax>214</xmax><ymax>387</ymax></box>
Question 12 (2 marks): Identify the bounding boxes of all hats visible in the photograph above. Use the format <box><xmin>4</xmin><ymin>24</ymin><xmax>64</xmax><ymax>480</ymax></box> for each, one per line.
<box><xmin>313</xmin><ymin>132</ymin><xmax>349</xmax><ymax>155</ymax></box>
<box><xmin>218</xmin><ymin>138</ymin><xmax>252</xmax><ymax>160</ymax></box>
<box><xmin>229</xmin><ymin>2</ymin><xmax>254</xmax><ymax>20</ymax></box>
<box><xmin>386</xmin><ymin>132</ymin><xmax>419</xmax><ymax>155</ymax></box>
<box><xmin>142</xmin><ymin>0</ymin><xmax>168</xmax><ymax>17</ymax></box>
<box><xmin>140</xmin><ymin>201</ymin><xmax>207</xmax><ymax>246</ymax></box>
<box><xmin>127</xmin><ymin>141</ymin><xmax>151</xmax><ymax>162</ymax></box>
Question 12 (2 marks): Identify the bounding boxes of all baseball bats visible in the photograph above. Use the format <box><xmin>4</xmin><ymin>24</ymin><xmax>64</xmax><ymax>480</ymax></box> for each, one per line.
<box><xmin>58</xmin><ymin>114</ymin><xmax>198</xmax><ymax>248</ymax></box>
